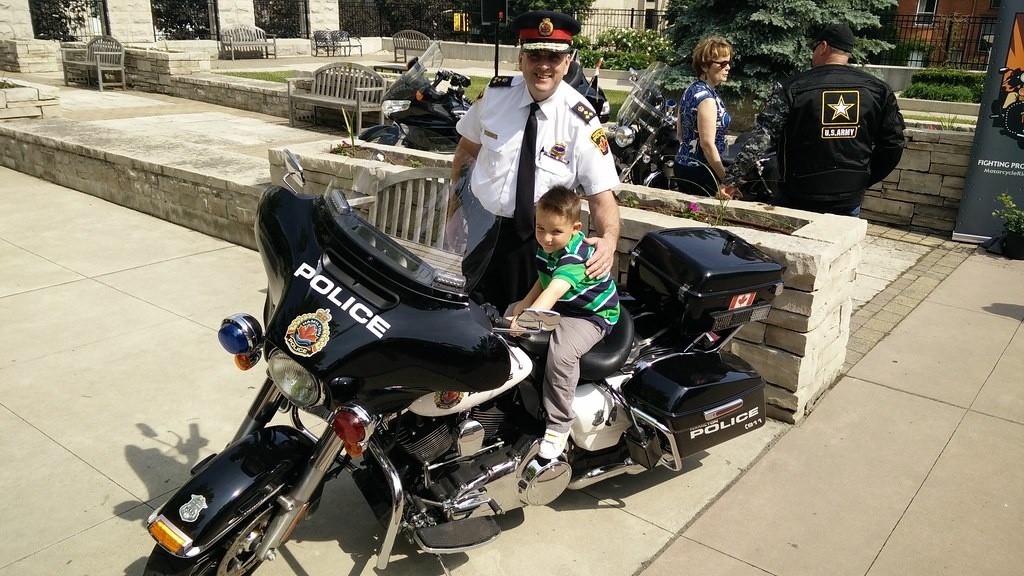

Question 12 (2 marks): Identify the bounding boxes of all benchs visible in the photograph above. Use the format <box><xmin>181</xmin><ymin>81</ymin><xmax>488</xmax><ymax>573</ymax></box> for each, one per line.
<box><xmin>347</xmin><ymin>167</ymin><xmax>465</xmax><ymax>293</ymax></box>
<box><xmin>219</xmin><ymin>24</ymin><xmax>277</xmax><ymax>62</ymax></box>
<box><xmin>284</xmin><ymin>61</ymin><xmax>387</xmax><ymax>138</ymax></box>
<box><xmin>392</xmin><ymin>29</ymin><xmax>444</xmax><ymax>68</ymax></box>
<box><xmin>58</xmin><ymin>34</ymin><xmax>126</xmax><ymax>92</ymax></box>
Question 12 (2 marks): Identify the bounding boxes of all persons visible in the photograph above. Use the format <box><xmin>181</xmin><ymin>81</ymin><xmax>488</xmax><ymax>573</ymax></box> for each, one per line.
<box><xmin>717</xmin><ymin>24</ymin><xmax>905</xmax><ymax>217</ymax></box>
<box><xmin>510</xmin><ymin>186</ymin><xmax>620</xmax><ymax>459</ymax></box>
<box><xmin>674</xmin><ymin>37</ymin><xmax>730</xmax><ymax>196</ymax></box>
<box><xmin>451</xmin><ymin>12</ymin><xmax>620</xmax><ymax>316</ymax></box>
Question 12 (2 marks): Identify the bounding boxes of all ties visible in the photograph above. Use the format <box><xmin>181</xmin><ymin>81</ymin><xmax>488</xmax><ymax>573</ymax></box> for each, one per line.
<box><xmin>514</xmin><ymin>103</ymin><xmax>541</xmax><ymax>241</ymax></box>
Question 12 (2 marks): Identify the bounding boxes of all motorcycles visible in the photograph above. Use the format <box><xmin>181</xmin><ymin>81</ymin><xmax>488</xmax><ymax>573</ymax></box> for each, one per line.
<box><xmin>596</xmin><ymin>56</ymin><xmax>778</xmax><ymax>198</ymax></box>
<box><xmin>144</xmin><ymin>122</ymin><xmax>787</xmax><ymax>576</ymax></box>
<box><xmin>359</xmin><ymin>39</ymin><xmax>606</xmax><ymax>154</ymax></box>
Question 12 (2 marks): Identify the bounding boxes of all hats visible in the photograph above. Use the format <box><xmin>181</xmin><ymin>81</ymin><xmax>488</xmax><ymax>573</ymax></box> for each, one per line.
<box><xmin>511</xmin><ymin>10</ymin><xmax>583</xmax><ymax>53</ymax></box>
<box><xmin>806</xmin><ymin>23</ymin><xmax>855</xmax><ymax>53</ymax></box>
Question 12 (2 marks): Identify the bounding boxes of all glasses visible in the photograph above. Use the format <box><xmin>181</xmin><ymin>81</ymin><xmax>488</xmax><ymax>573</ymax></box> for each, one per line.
<box><xmin>712</xmin><ymin>60</ymin><xmax>734</xmax><ymax>68</ymax></box>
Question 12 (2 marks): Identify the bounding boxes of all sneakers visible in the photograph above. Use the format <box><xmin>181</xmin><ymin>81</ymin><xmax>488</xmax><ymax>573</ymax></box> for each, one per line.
<box><xmin>538</xmin><ymin>427</ymin><xmax>572</xmax><ymax>460</ymax></box>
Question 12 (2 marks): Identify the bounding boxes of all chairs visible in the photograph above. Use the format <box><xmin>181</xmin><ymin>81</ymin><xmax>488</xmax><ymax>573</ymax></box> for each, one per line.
<box><xmin>330</xmin><ymin>30</ymin><xmax>363</xmax><ymax>57</ymax></box>
<box><xmin>313</xmin><ymin>29</ymin><xmax>347</xmax><ymax>57</ymax></box>
